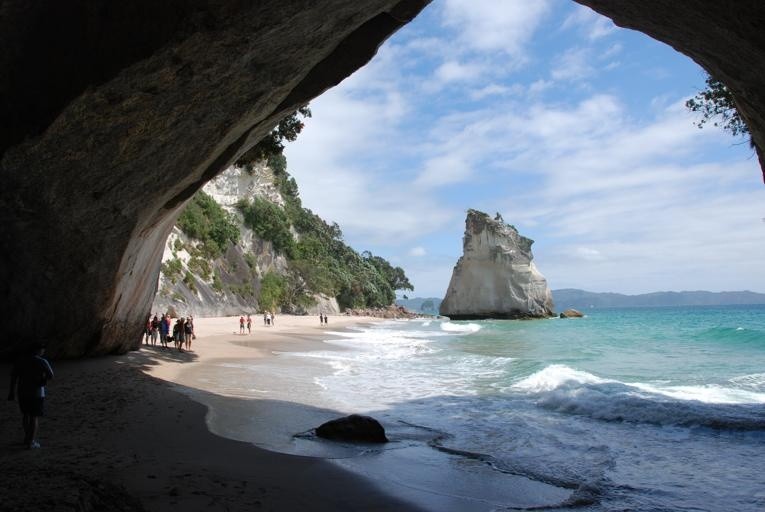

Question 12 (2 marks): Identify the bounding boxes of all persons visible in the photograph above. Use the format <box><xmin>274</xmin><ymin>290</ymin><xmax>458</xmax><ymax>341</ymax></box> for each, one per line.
<box><xmin>240</xmin><ymin>316</ymin><xmax>246</xmax><ymax>334</ymax></box>
<box><xmin>145</xmin><ymin>312</ymin><xmax>196</xmax><ymax>353</ymax></box>
<box><xmin>324</xmin><ymin>314</ymin><xmax>328</xmax><ymax>327</ymax></box>
<box><xmin>7</xmin><ymin>341</ymin><xmax>55</xmax><ymax>450</ymax></box>
<box><xmin>263</xmin><ymin>310</ymin><xmax>275</xmax><ymax>326</ymax></box>
<box><xmin>246</xmin><ymin>314</ymin><xmax>252</xmax><ymax>335</ymax></box>
<box><xmin>320</xmin><ymin>313</ymin><xmax>323</xmax><ymax>326</ymax></box>
